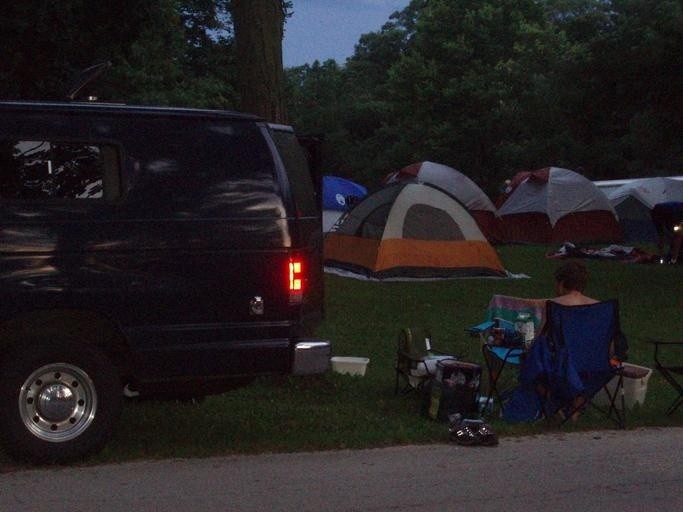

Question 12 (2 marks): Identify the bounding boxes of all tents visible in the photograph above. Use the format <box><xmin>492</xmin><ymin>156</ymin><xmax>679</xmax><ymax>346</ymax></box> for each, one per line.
<box><xmin>380</xmin><ymin>161</ymin><xmax>508</xmax><ymax>246</ymax></box>
<box><xmin>605</xmin><ymin>176</ymin><xmax>682</xmax><ymax>241</ymax></box>
<box><xmin>322</xmin><ymin>174</ymin><xmax>369</xmax><ymax>237</ymax></box>
<box><xmin>493</xmin><ymin>170</ymin><xmax>529</xmax><ymax>211</ymax></box>
<box><xmin>497</xmin><ymin>166</ymin><xmax>626</xmax><ymax>247</ymax></box>
<box><xmin>322</xmin><ymin>177</ymin><xmax>511</xmax><ymax>279</ymax></box>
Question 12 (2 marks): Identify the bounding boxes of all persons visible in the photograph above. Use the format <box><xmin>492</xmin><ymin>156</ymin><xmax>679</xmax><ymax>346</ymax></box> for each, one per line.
<box><xmin>668</xmin><ymin>222</ymin><xmax>682</xmax><ymax>264</ymax></box>
<box><xmin>623</xmin><ymin>237</ymin><xmax>680</xmax><ymax>266</ymax></box>
<box><xmin>543</xmin><ymin>242</ymin><xmax>607</xmax><ymax>260</ymax></box>
<box><xmin>522</xmin><ymin>259</ymin><xmax>613</xmax><ymax>424</ymax></box>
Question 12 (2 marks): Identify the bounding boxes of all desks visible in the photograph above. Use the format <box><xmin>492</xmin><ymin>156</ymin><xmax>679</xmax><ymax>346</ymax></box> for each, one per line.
<box><xmin>644</xmin><ymin>339</ymin><xmax>683</xmax><ymax>417</ymax></box>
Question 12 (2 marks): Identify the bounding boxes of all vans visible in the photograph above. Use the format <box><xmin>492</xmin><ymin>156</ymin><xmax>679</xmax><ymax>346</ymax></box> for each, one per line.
<box><xmin>0</xmin><ymin>98</ymin><xmax>332</xmax><ymax>469</ymax></box>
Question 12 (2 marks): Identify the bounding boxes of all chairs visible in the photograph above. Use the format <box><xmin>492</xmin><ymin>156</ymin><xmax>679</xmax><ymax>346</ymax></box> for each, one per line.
<box><xmin>394</xmin><ymin>294</ymin><xmax>629</xmax><ymax>431</ymax></box>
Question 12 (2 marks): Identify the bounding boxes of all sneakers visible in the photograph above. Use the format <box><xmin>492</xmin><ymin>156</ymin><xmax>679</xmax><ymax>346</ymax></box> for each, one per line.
<box><xmin>447</xmin><ymin>424</ymin><xmax>480</xmax><ymax>445</ymax></box>
<box><xmin>469</xmin><ymin>423</ymin><xmax>499</xmax><ymax>445</ymax></box>
